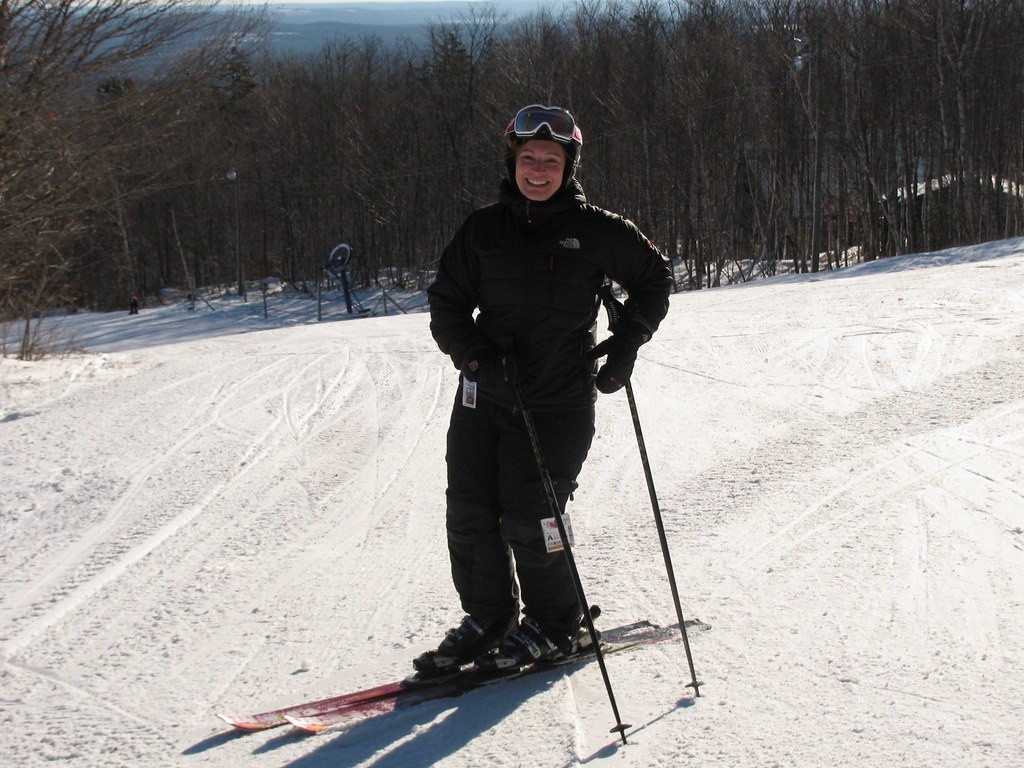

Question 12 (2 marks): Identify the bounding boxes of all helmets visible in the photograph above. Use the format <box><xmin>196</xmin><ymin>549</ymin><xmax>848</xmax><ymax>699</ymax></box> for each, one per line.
<box><xmin>505</xmin><ymin>118</ymin><xmax>583</xmax><ymax>187</ymax></box>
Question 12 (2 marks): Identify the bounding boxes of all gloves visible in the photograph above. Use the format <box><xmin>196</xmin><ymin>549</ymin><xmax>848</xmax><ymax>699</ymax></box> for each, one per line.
<box><xmin>448</xmin><ymin>336</ymin><xmax>521</xmax><ymax>387</ymax></box>
<box><xmin>584</xmin><ymin>322</ymin><xmax>653</xmax><ymax>394</ymax></box>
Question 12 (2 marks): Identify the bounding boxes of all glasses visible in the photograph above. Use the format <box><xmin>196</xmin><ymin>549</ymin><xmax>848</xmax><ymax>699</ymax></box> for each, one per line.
<box><xmin>514</xmin><ymin>105</ymin><xmax>576</xmax><ymax>142</ymax></box>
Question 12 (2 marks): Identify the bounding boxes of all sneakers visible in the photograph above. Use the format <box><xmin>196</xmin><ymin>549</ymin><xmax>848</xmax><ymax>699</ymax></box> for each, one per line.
<box><xmin>403</xmin><ymin>617</ymin><xmax>603</xmax><ymax>689</ymax></box>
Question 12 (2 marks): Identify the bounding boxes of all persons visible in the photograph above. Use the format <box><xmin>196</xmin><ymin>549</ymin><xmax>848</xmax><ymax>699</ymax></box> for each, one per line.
<box><xmin>412</xmin><ymin>104</ymin><xmax>674</xmax><ymax>682</ymax></box>
<box><xmin>129</xmin><ymin>296</ymin><xmax>139</xmax><ymax>314</ymax></box>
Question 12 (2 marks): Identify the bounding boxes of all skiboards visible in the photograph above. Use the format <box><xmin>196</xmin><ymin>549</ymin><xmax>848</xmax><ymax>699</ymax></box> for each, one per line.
<box><xmin>213</xmin><ymin>616</ymin><xmax>714</xmax><ymax>734</ymax></box>
